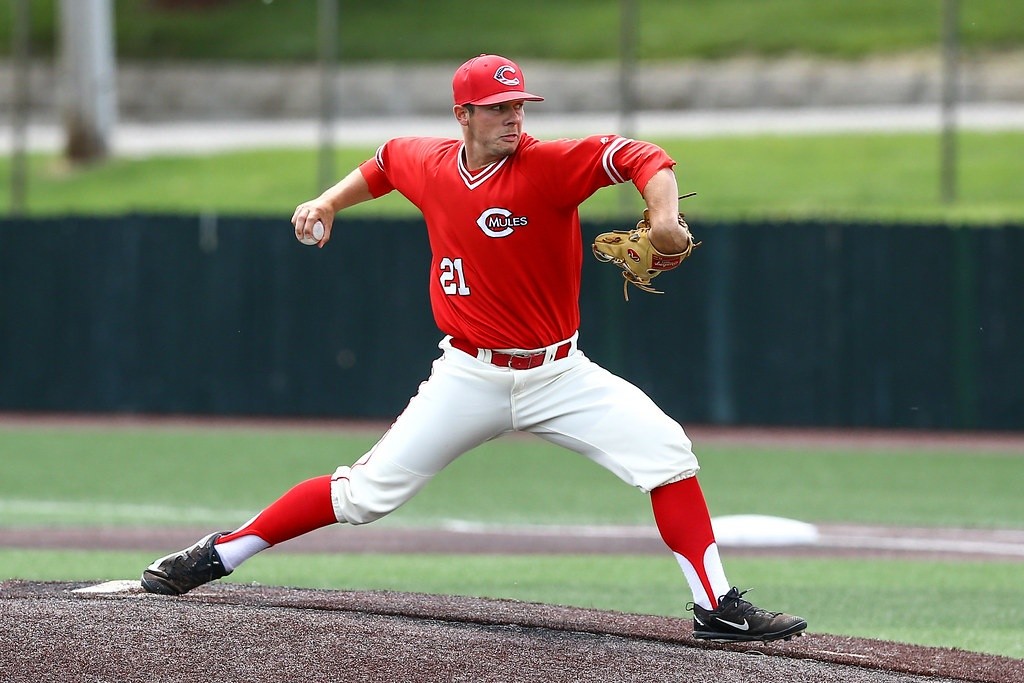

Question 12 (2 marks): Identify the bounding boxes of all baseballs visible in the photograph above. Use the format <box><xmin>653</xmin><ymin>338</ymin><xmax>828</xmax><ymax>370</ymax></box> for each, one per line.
<box><xmin>295</xmin><ymin>219</ymin><xmax>324</xmax><ymax>245</ymax></box>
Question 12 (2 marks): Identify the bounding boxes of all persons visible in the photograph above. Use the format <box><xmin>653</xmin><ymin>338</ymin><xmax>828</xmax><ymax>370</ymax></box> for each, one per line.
<box><xmin>142</xmin><ymin>52</ymin><xmax>808</xmax><ymax>642</ymax></box>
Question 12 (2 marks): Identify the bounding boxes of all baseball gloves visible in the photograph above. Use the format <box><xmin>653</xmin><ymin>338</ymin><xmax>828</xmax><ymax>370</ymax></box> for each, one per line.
<box><xmin>594</xmin><ymin>208</ymin><xmax>693</xmax><ymax>283</ymax></box>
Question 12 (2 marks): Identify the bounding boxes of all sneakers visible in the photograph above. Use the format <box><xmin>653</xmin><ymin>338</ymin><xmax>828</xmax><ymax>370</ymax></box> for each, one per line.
<box><xmin>141</xmin><ymin>530</ymin><xmax>236</xmax><ymax>595</ymax></box>
<box><xmin>685</xmin><ymin>587</ymin><xmax>807</xmax><ymax>642</ymax></box>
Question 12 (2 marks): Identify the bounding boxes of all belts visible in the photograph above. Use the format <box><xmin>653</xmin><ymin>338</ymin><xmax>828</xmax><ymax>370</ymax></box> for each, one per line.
<box><xmin>450</xmin><ymin>337</ymin><xmax>571</xmax><ymax>370</ymax></box>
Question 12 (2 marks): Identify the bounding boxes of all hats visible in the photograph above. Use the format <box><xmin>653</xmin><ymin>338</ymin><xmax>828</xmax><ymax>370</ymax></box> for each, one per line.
<box><xmin>453</xmin><ymin>53</ymin><xmax>544</xmax><ymax>107</ymax></box>
